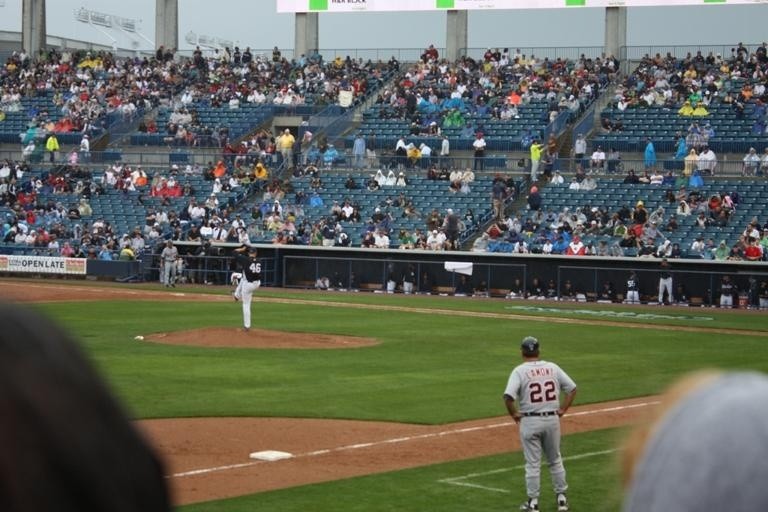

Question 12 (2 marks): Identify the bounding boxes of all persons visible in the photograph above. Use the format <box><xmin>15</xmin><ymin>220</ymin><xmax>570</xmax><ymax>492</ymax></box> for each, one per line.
<box><xmin>503</xmin><ymin>336</ymin><xmax>576</xmax><ymax>512</ymax></box>
<box><xmin>230</xmin><ymin>245</ymin><xmax>263</xmax><ymax>332</ymax></box>
<box><xmin>1</xmin><ymin>39</ymin><xmax>768</xmax><ymax>311</ymax></box>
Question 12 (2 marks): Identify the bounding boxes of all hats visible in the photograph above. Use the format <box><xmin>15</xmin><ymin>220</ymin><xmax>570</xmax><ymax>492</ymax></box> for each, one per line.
<box><xmin>521</xmin><ymin>335</ymin><xmax>540</xmax><ymax>352</ymax></box>
<box><xmin>247</xmin><ymin>247</ymin><xmax>257</xmax><ymax>254</ymax></box>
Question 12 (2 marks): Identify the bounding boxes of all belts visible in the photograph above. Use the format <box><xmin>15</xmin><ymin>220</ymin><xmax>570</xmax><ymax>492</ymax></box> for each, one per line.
<box><xmin>521</xmin><ymin>412</ymin><xmax>559</xmax><ymax>417</ymax></box>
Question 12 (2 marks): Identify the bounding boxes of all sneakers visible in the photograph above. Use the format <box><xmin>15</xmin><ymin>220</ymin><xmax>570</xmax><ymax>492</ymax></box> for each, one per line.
<box><xmin>521</xmin><ymin>497</ymin><xmax>539</xmax><ymax>511</ymax></box>
<box><xmin>556</xmin><ymin>494</ymin><xmax>570</xmax><ymax>511</ymax></box>
